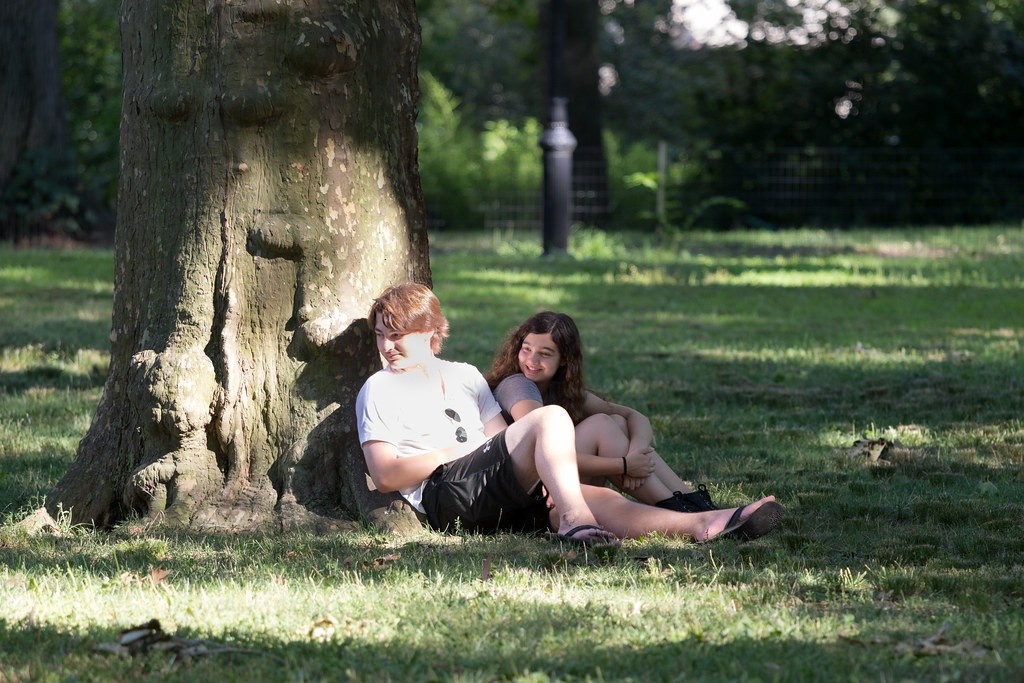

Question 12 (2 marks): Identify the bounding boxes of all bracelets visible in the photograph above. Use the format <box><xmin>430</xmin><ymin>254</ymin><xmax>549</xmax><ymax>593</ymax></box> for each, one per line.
<box><xmin>623</xmin><ymin>456</ymin><xmax>629</xmax><ymax>477</ymax></box>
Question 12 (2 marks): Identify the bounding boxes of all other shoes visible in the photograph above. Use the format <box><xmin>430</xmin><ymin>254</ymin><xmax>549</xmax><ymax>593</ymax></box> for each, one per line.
<box><xmin>654</xmin><ymin>484</ymin><xmax>720</xmax><ymax>513</ymax></box>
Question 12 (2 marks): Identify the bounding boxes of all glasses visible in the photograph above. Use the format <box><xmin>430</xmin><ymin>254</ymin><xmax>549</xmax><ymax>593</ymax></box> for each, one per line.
<box><xmin>445</xmin><ymin>408</ymin><xmax>467</xmax><ymax>443</ymax></box>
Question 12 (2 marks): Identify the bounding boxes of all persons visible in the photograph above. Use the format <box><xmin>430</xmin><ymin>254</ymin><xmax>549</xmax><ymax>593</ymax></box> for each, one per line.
<box><xmin>355</xmin><ymin>281</ymin><xmax>786</xmax><ymax>545</ymax></box>
<box><xmin>483</xmin><ymin>311</ymin><xmax>722</xmax><ymax>512</ymax></box>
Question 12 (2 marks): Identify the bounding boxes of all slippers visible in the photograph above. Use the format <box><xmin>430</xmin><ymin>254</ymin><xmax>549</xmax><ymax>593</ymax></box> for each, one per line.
<box><xmin>544</xmin><ymin>525</ymin><xmax>621</xmax><ymax>549</ymax></box>
<box><xmin>694</xmin><ymin>501</ymin><xmax>786</xmax><ymax>545</ymax></box>
